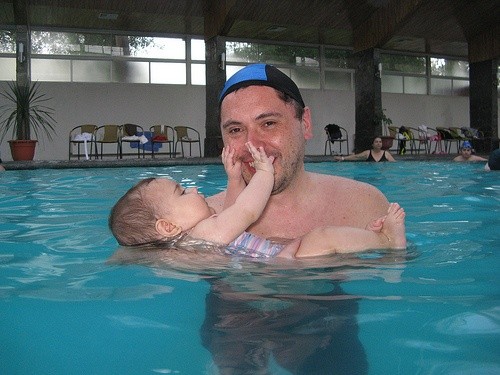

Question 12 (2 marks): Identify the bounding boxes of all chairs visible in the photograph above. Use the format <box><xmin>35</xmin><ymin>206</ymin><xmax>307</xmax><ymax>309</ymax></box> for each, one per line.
<box><xmin>68</xmin><ymin>124</ymin><xmax>202</xmax><ymax>160</ymax></box>
<box><xmin>387</xmin><ymin>125</ymin><xmax>484</xmax><ymax>156</ymax></box>
<box><xmin>324</xmin><ymin>124</ymin><xmax>349</xmax><ymax>156</ymax></box>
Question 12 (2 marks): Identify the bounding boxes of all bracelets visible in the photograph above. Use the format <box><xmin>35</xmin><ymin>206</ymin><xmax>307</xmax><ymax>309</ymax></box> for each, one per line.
<box><xmin>342</xmin><ymin>157</ymin><xmax>345</xmax><ymax>162</ymax></box>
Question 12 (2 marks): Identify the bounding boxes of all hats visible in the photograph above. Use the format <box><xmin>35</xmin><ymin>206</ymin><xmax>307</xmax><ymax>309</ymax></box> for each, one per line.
<box><xmin>462</xmin><ymin>141</ymin><xmax>472</xmax><ymax>148</ymax></box>
<box><xmin>219</xmin><ymin>64</ymin><xmax>304</xmax><ymax>110</ymax></box>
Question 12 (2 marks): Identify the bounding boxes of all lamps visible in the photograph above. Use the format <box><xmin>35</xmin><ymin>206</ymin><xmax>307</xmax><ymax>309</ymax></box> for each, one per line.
<box><xmin>18</xmin><ymin>43</ymin><xmax>25</xmax><ymax>63</ymax></box>
<box><xmin>375</xmin><ymin>63</ymin><xmax>382</xmax><ymax>78</ymax></box>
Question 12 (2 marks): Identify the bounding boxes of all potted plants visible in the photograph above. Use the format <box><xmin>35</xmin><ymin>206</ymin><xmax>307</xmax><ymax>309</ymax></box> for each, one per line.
<box><xmin>0</xmin><ymin>80</ymin><xmax>57</xmax><ymax>163</ymax></box>
<box><xmin>381</xmin><ymin>108</ymin><xmax>394</xmax><ymax>150</ymax></box>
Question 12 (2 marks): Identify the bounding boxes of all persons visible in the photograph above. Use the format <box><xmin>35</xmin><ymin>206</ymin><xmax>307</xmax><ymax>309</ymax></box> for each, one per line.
<box><xmin>454</xmin><ymin>141</ymin><xmax>500</xmax><ymax>171</ymax></box>
<box><xmin>107</xmin><ymin>61</ymin><xmax>406</xmax><ymax>266</ymax></box>
